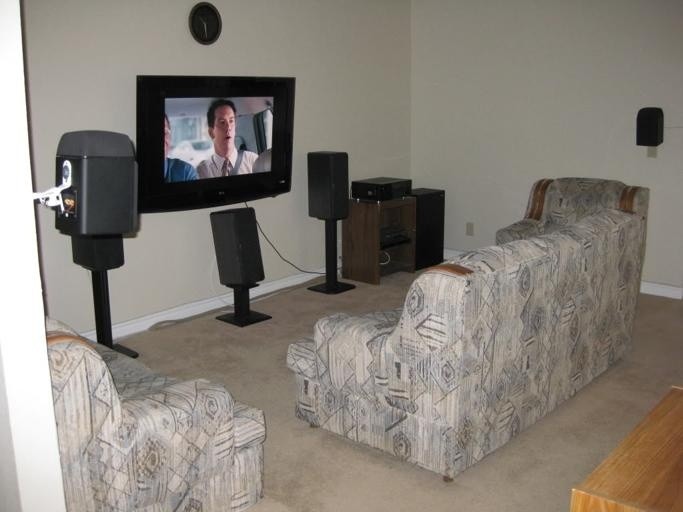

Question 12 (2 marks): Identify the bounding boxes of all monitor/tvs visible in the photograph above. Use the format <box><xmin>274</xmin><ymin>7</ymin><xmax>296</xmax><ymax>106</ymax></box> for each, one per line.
<box><xmin>136</xmin><ymin>75</ymin><xmax>295</xmax><ymax>214</ymax></box>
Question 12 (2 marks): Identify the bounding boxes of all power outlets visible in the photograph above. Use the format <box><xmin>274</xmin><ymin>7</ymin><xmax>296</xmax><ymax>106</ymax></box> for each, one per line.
<box><xmin>466</xmin><ymin>223</ymin><xmax>474</xmax><ymax>236</ymax></box>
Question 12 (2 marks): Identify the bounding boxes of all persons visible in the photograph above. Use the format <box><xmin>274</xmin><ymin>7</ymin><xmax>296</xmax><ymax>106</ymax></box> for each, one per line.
<box><xmin>195</xmin><ymin>99</ymin><xmax>258</xmax><ymax>179</ymax></box>
<box><xmin>164</xmin><ymin>112</ymin><xmax>198</xmax><ymax>182</ymax></box>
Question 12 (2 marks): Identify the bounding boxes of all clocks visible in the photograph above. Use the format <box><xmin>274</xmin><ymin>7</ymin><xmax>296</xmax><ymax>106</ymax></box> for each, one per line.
<box><xmin>189</xmin><ymin>2</ymin><xmax>224</xmax><ymax>47</ymax></box>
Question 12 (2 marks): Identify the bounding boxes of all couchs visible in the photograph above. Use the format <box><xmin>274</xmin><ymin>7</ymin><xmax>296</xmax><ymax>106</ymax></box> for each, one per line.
<box><xmin>46</xmin><ymin>315</ymin><xmax>266</xmax><ymax>512</ymax></box>
<box><xmin>285</xmin><ymin>178</ymin><xmax>650</xmax><ymax>480</ymax></box>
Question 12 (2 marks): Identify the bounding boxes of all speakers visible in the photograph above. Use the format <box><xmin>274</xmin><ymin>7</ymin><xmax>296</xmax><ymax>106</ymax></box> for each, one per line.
<box><xmin>308</xmin><ymin>152</ymin><xmax>350</xmax><ymax>223</ymax></box>
<box><xmin>208</xmin><ymin>207</ymin><xmax>266</xmax><ymax>288</ymax></box>
<box><xmin>71</xmin><ymin>235</ymin><xmax>124</xmax><ymax>270</ymax></box>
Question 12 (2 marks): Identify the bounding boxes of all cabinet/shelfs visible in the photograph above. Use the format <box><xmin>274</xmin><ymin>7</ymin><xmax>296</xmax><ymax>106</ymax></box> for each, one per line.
<box><xmin>342</xmin><ymin>188</ymin><xmax>445</xmax><ymax>285</ymax></box>
